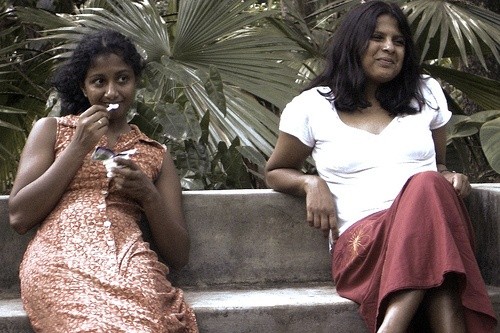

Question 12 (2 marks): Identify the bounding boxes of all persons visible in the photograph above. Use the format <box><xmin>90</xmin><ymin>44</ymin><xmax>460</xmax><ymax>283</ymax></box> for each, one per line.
<box><xmin>265</xmin><ymin>1</ymin><xmax>497</xmax><ymax>333</ymax></box>
<box><xmin>8</xmin><ymin>27</ymin><xmax>199</xmax><ymax>333</ymax></box>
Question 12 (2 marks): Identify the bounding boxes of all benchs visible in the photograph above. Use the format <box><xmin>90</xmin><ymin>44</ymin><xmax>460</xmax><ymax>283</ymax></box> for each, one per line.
<box><xmin>0</xmin><ymin>183</ymin><xmax>500</xmax><ymax>333</ymax></box>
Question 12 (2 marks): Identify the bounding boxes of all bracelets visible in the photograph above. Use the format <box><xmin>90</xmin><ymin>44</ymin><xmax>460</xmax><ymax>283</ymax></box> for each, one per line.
<box><xmin>439</xmin><ymin>169</ymin><xmax>454</xmax><ymax>174</ymax></box>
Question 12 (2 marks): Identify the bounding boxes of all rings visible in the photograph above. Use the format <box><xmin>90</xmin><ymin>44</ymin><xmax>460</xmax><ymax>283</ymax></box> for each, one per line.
<box><xmin>98</xmin><ymin>120</ymin><xmax>103</xmax><ymax>127</ymax></box>
